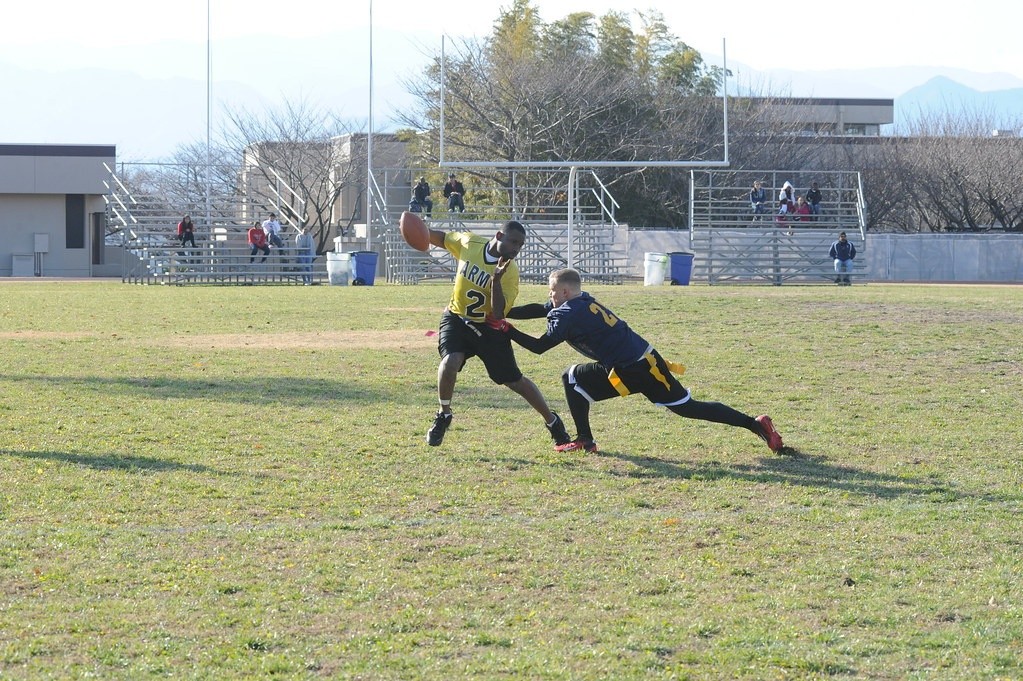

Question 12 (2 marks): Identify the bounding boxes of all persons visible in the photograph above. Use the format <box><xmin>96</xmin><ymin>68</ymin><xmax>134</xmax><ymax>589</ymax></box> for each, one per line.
<box><xmin>399</xmin><ymin>219</ymin><xmax>570</xmax><ymax>446</ymax></box>
<box><xmin>408</xmin><ymin>176</ymin><xmax>433</xmax><ymax>219</ymax></box>
<box><xmin>828</xmin><ymin>232</ymin><xmax>857</xmax><ymax>283</ymax></box>
<box><xmin>485</xmin><ymin>268</ymin><xmax>783</xmax><ymax>451</ymax></box>
<box><xmin>750</xmin><ymin>180</ymin><xmax>767</xmax><ymax>219</ymax></box>
<box><xmin>775</xmin><ymin>181</ymin><xmax>822</xmax><ymax>228</ymax></box>
<box><xmin>262</xmin><ymin>213</ymin><xmax>283</xmax><ymax>248</ymax></box>
<box><xmin>443</xmin><ymin>173</ymin><xmax>467</xmax><ymax>213</ymax></box>
<box><xmin>248</xmin><ymin>221</ymin><xmax>270</xmax><ymax>265</ymax></box>
<box><xmin>295</xmin><ymin>227</ymin><xmax>316</xmax><ymax>285</ymax></box>
<box><xmin>177</xmin><ymin>214</ymin><xmax>199</xmax><ymax>248</ymax></box>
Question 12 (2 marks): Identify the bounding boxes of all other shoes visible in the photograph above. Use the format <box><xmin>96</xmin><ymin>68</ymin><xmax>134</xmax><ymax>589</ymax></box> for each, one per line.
<box><xmin>835</xmin><ymin>278</ymin><xmax>841</xmax><ymax>283</ymax></box>
<box><xmin>193</xmin><ymin>246</ymin><xmax>198</xmax><ymax>248</ymax></box>
<box><xmin>425</xmin><ymin>216</ymin><xmax>431</xmax><ymax>220</ymax></box>
<box><xmin>843</xmin><ymin>278</ymin><xmax>850</xmax><ymax>282</ymax></box>
<box><xmin>448</xmin><ymin>208</ymin><xmax>453</xmax><ymax>212</ymax></box>
<box><xmin>462</xmin><ymin>209</ymin><xmax>467</xmax><ymax>213</ymax></box>
<box><xmin>268</xmin><ymin>244</ymin><xmax>274</xmax><ymax>248</ymax></box>
<box><xmin>181</xmin><ymin>245</ymin><xmax>185</xmax><ymax>248</ymax></box>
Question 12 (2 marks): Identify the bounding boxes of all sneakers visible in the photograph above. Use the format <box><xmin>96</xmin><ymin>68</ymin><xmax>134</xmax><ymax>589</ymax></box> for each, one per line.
<box><xmin>754</xmin><ymin>415</ymin><xmax>784</xmax><ymax>453</ymax></box>
<box><xmin>545</xmin><ymin>412</ymin><xmax>570</xmax><ymax>445</ymax></box>
<box><xmin>553</xmin><ymin>437</ymin><xmax>597</xmax><ymax>454</ymax></box>
<box><xmin>428</xmin><ymin>408</ymin><xmax>452</xmax><ymax>446</ymax></box>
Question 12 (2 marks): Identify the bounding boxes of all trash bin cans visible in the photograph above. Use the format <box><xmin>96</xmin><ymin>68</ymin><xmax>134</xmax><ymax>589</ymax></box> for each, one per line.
<box><xmin>349</xmin><ymin>251</ymin><xmax>378</xmax><ymax>286</ymax></box>
<box><xmin>643</xmin><ymin>252</ymin><xmax>668</xmax><ymax>286</ymax></box>
<box><xmin>667</xmin><ymin>252</ymin><xmax>694</xmax><ymax>286</ymax></box>
<box><xmin>326</xmin><ymin>251</ymin><xmax>351</xmax><ymax>286</ymax></box>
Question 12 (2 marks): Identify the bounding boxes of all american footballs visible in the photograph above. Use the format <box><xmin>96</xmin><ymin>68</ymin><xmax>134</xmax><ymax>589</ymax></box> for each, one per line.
<box><xmin>400</xmin><ymin>210</ymin><xmax>431</xmax><ymax>252</ymax></box>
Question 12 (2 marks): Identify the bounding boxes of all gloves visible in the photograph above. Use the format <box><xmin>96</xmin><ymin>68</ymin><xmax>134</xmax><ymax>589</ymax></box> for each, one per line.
<box><xmin>485</xmin><ymin>313</ymin><xmax>510</xmax><ymax>334</ymax></box>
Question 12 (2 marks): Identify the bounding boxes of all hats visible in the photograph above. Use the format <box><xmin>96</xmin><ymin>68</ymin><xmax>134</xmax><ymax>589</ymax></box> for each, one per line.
<box><xmin>839</xmin><ymin>232</ymin><xmax>847</xmax><ymax>238</ymax></box>
<box><xmin>449</xmin><ymin>173</ymin><xmax>455</xmax><ymax>178</ymax></box>
